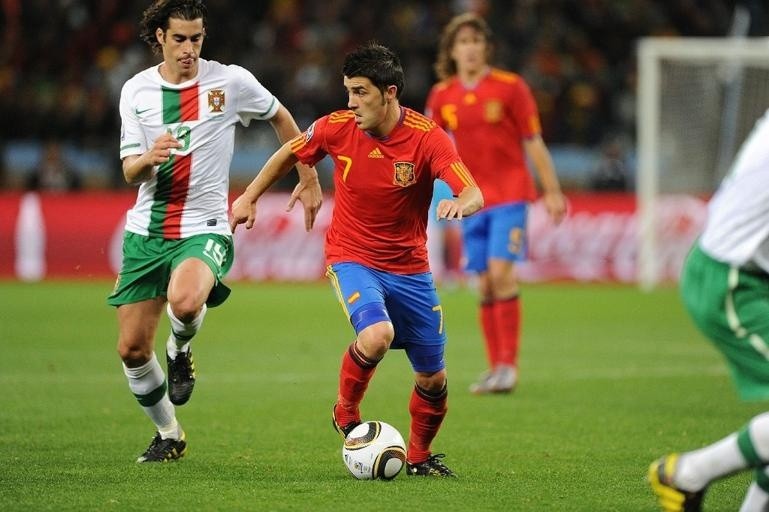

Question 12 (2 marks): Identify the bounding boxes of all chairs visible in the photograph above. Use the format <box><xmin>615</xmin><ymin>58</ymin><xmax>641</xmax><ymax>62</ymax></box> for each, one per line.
<box><xmin>469</xmin><ymin>365</ymin><xmax>517</xmax><ymax>394</ymax></box>
<box><xmin>166</xmin><ymin>345</ymin><xmax>196</xmax><ymax>405</ymax></box>
<box><xmin>406</xmin><ymin>454</ymin><xmax>456</xmax><ymax>477</ymax></box>
<box><xmin>137</xmin><ymin>431</ymin><xmax>186</xmax><ymax>462</ymax></box>
<box><xmin>648</xmin><ymin>454</ymin><xmax>704</xmax><ymax>512</ymax></box>
<box><xmin>332</xmin><ymin>404</ymin><xmax>362</xmax><ymax>440</ymax></box>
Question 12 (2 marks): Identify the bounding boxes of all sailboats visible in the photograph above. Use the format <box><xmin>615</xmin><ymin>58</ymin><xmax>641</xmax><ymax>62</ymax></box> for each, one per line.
<box><xmin>342</xmin><ymin>421</ymin><xmax>409</xmax><ymax>481</ymax></box>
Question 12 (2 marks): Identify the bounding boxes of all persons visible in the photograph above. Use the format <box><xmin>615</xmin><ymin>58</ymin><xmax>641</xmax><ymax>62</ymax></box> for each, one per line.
<box><xmin>105</xmin><ymin>0</ymin><xmax>323</xmax><ymax>472</ymax></box>
<box><xmin>643</xmin><ymin>106</ymin><xmax>769</xmax><ymax>511</ymax></box>
<box><xmin>0</xmin><ymin>0</ymin><xmax>165</xmax><ymax>195</ymax></box>
<box><xmin>200</xmin><ymin>1</ymin><xmax>459</xmax><ymax>196</ymax></box>
<box><xmin>227</xmin><ymin>38</ymin><xmax>485</xmax><ymax>479</ymax></box>
<box><xmin>459</xmin><ymin>0</ymin><xmax>769</xmax><ymax>195</ymax></box>
<box><xmin>423</xmin><ymin>11</ymin><xmax>569</xmax><ymax>397</ymax></box>
